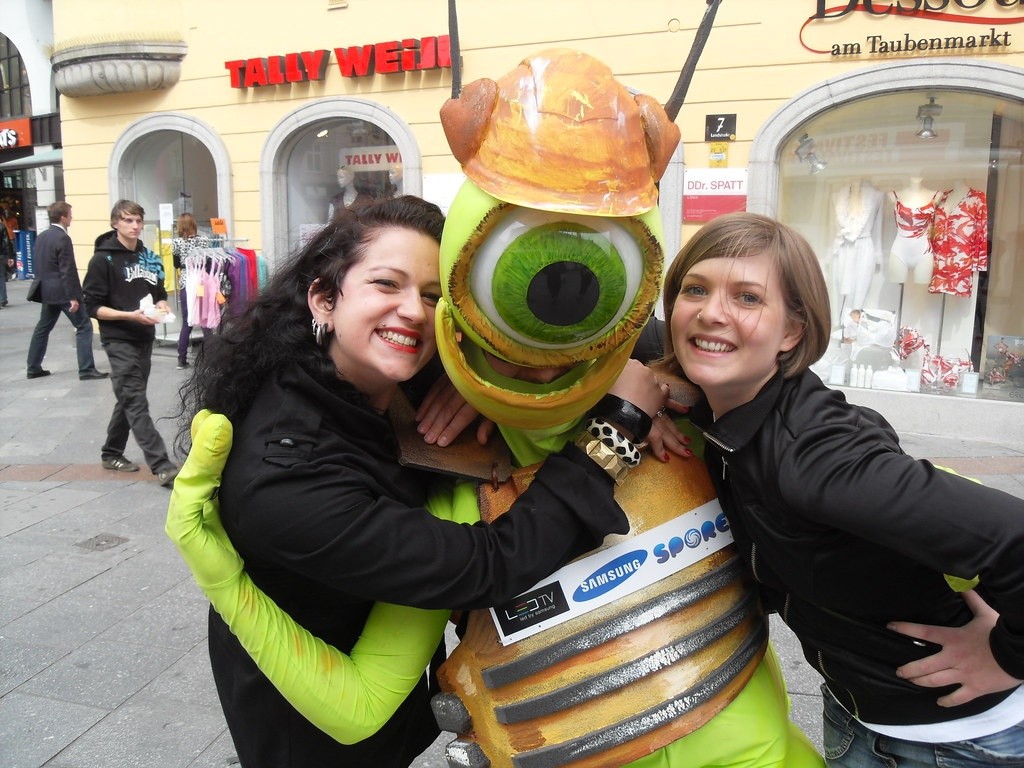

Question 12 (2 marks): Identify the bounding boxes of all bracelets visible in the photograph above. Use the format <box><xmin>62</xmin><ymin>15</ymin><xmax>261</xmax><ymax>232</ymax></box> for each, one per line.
<box><xmin>576</xmin><ymin>432</ymin><xmax>631</xmax><ymax>488</ymax></box>
<box><xmin>585</xmin><ymin>418</ymin><xmax>642</xmax><ymax>468</ymax></box>
<box><xmin>587</xmin><ymin>394</ymin><xmax>653</xmax><ymax>444</ymax></box>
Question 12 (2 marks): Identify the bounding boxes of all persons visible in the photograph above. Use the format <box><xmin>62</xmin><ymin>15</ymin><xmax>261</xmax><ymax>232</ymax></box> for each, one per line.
<box><xmin>830</xmin><ymin>177</ymin><xmax>988</xmax><ymax>343</ymax></box>
<box><xmin>165</xmin><ymin>49</ymin><xmax>986</xmax><ymax>768</ymax></box>
<box><xmin>415</xmin><ymin>215</ymin><xmax>1024</xmax><ymax>768</ymax></box>
<box><xmin>327</xmin><ymin>169</ymin><xmax>365</xmax><ymax>228</ymax></box>
<box><xmin>187</xmin><ymin>196</ymin><xmax>692</xmax><ymax>768</ymax></box>
<box><xmin>27</xmin><ymin>203</ymin><xmax>109</xmax><ymax>380</ymax></box>
<box><xmin>0</xmin><ymin>223</ymin><xmax>14</xmax><ymax>306</ymax></box>
<box><xmin>176</xmin><ymin>212</ymin><xmax>213</xmax><ymax>368</ymax></box>
<box><xmin>388</xmin><ymin>163</ymin><xmax>403</xmax><ymax>198</ymax></box>
<box><xmin>82</xmin><ymin>200</ymin><xmax>179</xmax><ymax>487</ymax></box>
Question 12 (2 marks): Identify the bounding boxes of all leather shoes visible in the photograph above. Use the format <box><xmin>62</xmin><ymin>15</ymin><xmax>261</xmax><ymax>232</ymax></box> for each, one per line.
<box><xmin>27</xmin><ymin>369</ymin><xmax>51</xmax><ymax>379</ymax></box>
<box><xmin>79</xmin><ymin>370</ymin><xmax>109</xmax><ymax>380</ymax></box>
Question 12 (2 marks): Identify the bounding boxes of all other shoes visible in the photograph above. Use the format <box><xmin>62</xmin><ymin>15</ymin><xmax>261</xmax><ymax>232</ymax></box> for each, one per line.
<box><xmin>0</xmin><ymin>300</ymin><xmax>7</xmax><ymax>309</ymax></box>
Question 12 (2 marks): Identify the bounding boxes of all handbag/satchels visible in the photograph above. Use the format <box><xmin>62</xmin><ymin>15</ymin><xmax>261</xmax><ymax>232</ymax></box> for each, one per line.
<box><xmin>26</xmin><ymin>278</ymin><xmax>42</xmax><ymax>303</ymax></box>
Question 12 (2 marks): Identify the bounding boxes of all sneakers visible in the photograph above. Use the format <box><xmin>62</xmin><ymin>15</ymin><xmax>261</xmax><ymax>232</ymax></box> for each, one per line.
<box><xmin>158</xmin><ymin>464</ymin><xmax>178</xmax><ymax>486</ymax></box>
<box><xmin>101</xmin><ymin>454</ymin><xmax>139</xmax><ymax>472</ymax></box>
<box><xmin>177</xmin><ymin>361</ymin><xmax>189</xmax><ymax>370</ymax></box>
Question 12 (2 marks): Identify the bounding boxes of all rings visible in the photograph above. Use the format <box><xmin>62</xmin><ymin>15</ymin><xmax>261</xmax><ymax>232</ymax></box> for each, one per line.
<box><xmin>657</xmin><ymin>406</ymin><xmax>666</xmax><ymax>417</ymax></box>
